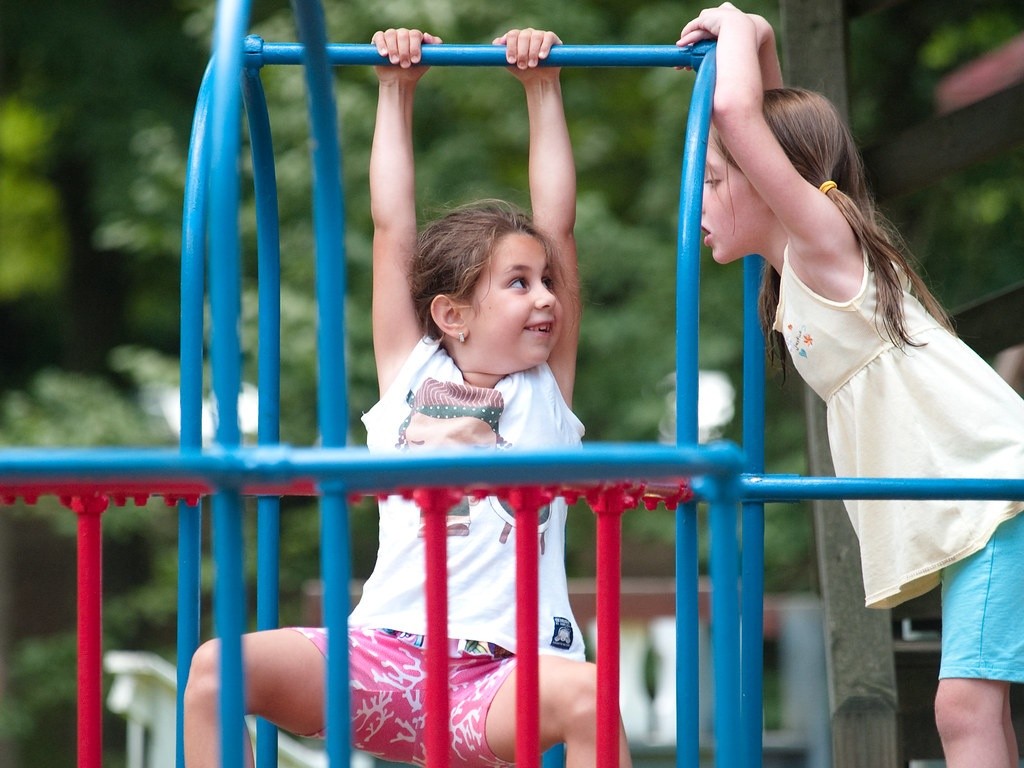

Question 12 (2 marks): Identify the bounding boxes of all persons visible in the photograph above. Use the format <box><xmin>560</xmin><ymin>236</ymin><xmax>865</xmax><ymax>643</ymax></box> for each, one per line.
<box><xmin>175</xmin><ymin>20</ymin><xmax>638</xmax><ymax>768</ymax></box>
<box><xmin>666</xmin><ymin>1</ymin><xmax>1024</xmax><ymax>768</ymax></box>
